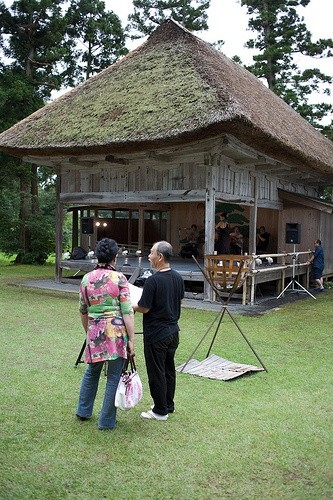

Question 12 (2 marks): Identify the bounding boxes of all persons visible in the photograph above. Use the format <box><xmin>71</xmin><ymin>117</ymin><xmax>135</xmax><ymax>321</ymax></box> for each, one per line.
<box><xmin>214</xmin><ymin>213</ymin><xmax>231</xmax><ymax>255</ymax></box>
<box><xmin>229</xmin><ymin>226</ymin><xmax>243</xmax><ymax>254</ymax></box>
<box><xmin>306</xmin><ymin>239</ymin><xmax>324</xmax><ymax>291</ymax></box>
<box><xmin>179</xmin><ymin>224</ymin><xmax>203</xmax><ymax>257</ymax></box>
<box><xmin>131</xmin><ymin>240</ymin><xmax>185</xmax><ymax>422</ymax></box>
<box><xmin>75</xmin><ymin>238</ymin><xmax>135</xmax><ymax>430</ymax></box>
<box><xmin>256</xmin><ymin>226</ymin><xmax>271</xmax><ymax>252</ymax></box>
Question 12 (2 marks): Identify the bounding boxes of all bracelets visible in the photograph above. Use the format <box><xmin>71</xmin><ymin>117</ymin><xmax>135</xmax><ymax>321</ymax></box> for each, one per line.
<box><xmin>308</xmin><ymin>260</ymin><xmax>312</xmax><ymax>263</ymax></box>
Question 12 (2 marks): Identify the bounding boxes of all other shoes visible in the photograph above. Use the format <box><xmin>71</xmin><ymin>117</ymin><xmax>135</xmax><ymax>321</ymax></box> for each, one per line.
<box><xmin>75</xmin><ymin>414</ymin><xmax>86</xmax><ymax>420</ymax></box>
<box><xmin>217</xmin><ymin>261</ymin><xmax>222</xmax><ymax>266</ymax></box>
<box><xmin>98</xmin><ymin>420</ymin><xmax>117</xmax><ymax>430</ymax></box>
<box><xmin>313</xmin><ymin>287</ymin><xmax>325</xmax><ymax>292</ymax></box>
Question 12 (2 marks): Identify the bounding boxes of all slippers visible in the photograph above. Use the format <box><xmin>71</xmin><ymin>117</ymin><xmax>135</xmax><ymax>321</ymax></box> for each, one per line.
<box><xmin>140</xmin><ymin>405</ymin><xmax>175</xmax><ymax>420</ymax></box>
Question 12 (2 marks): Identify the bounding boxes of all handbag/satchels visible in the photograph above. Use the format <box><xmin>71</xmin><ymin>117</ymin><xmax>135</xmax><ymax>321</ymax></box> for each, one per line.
<box><xmin>114</xmin><ymin>352</ymin><xmax>141</xmax><ymax>411</ymax></box>
